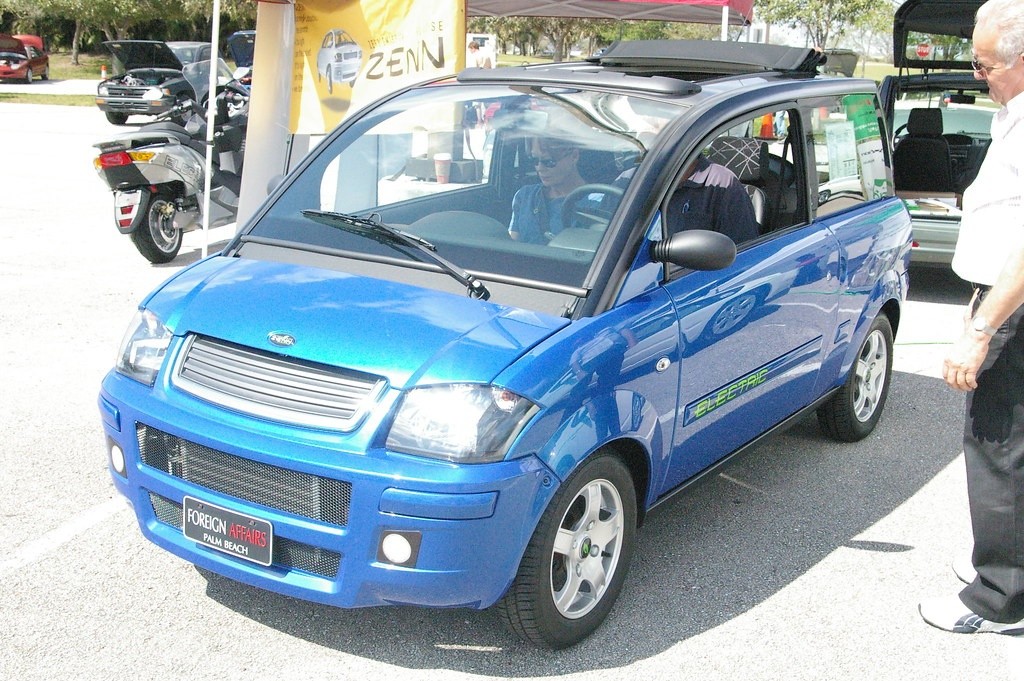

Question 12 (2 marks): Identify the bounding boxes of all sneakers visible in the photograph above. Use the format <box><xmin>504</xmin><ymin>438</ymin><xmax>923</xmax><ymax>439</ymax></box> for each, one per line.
<box><xmin>953</xmin><ymin>556</ymin><xmax>979</xmax><ymax>585</ymax></box>
<box><xmin>918</xmin><ymin>594</ymin><xmax>1024</xmax><ymax>636</ymax></box>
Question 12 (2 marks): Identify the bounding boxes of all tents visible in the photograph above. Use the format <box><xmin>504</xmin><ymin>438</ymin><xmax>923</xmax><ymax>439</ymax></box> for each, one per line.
<box><xmin>201</xmin><ymin>0</ymin><xmax>754</xmax><ymax>261</ymax></box>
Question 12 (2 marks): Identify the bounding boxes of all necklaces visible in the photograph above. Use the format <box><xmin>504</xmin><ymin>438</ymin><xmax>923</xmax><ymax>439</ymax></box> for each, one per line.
<box><xmin>533</xmin><ymin>195</ymin><xmax>561</xmax><ymax>214</ymax></box>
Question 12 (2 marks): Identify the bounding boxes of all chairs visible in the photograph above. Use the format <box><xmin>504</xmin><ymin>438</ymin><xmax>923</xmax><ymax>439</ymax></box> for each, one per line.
<box><xmin>894</xmin><ymin>107</ymin><xmax>955</xmax><ymax>192</ymax></box>
<box><xmin>573</xmin><ymin>132</ymin><xmax>620</xmax><ymax>188</ymax></box>
<box><xmin>706</xmin><ymin>135</ymin><xmax>772</xmax><ymax>237</ymax></box>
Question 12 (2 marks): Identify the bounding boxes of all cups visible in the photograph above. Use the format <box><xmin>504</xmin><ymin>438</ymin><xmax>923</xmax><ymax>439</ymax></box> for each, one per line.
<box><xmin>434</xmin><ymin>154</ymin><xmax>452</xmax><ymax>184</ymax></box>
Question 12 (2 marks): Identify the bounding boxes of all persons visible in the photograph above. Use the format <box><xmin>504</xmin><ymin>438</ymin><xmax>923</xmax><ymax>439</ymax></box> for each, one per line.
<box><xmin>915</xmin><ymin>0</ymin><xmax>1024</xmax><ymax>638</ymax></box>
<box><xmin>598</xmin><ymin>152</ymin><xmax>758</xmax><ymax>247</ymax></box>
<box><xmin>467</xmin><ymin>39</ymin><xmax>491</xmax><ymax>124</ymax></box>
<box><xmin>507</xmin><ymin>128</ymin><xmax>603</xmax><ymax>246</ymax></box>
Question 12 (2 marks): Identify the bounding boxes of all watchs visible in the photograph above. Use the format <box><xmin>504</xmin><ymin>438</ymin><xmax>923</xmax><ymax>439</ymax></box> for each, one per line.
<box><xmin>971</xmin><ymin>315</ymin><xmax>997</xmax><ymax>336</ymax></box>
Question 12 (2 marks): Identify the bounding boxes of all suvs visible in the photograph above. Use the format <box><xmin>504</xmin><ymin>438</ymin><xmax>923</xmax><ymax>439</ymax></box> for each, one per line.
<box><xmin>97</xmin><ymin>38</ymin><xmax>913</xmax><ymax>650</ymax></box>
<box><xmin>879</xmin><ymin>0</ymin><xmax>1001</xmax><ymax>265</ymax></box>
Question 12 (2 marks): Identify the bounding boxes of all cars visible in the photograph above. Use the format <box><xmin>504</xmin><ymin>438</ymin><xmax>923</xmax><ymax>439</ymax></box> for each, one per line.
<box><xmin>316</xmin><ymin>28</ymin><xmax>364</xmax><ymax>95</ymax></box>
<box><xmin>94</xmin><ymin>39</ymin><xmax>225</xmax><ymax>125</ymax></box>
<box><xmin>0</xmin><ymin>33</ymin><xmax>50</xmax><ymax>83</ymax></box>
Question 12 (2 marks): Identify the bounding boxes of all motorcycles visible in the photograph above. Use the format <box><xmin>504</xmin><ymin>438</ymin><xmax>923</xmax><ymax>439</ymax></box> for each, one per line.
<box><xmin>94</xmin><ymin>64</ymin><xmax>252</xmax><ymax>265</ymax></box>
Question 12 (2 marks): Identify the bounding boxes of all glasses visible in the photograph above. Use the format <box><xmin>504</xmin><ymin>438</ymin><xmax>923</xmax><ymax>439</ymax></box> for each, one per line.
<box><xmin>529</xmin><ymin>158</ymin><xmax>559</xmax><ymax>167</ymax></box>
<box><xmin>970</xmin><ymin>48</ymin><xmax>1002</xmax><ymax>72</ymax></box>
